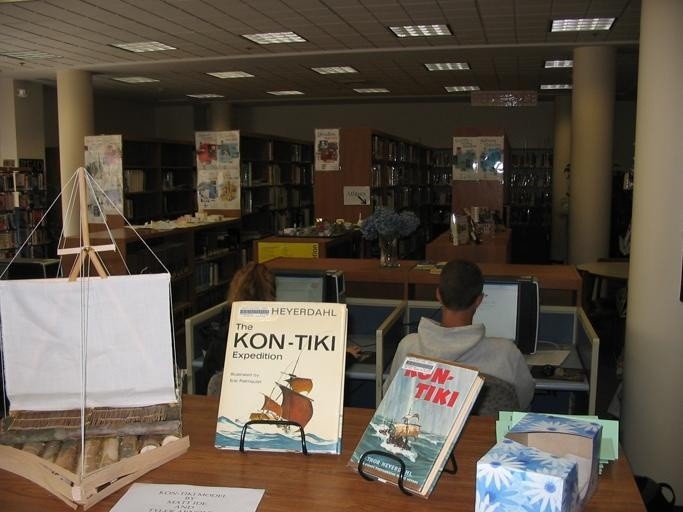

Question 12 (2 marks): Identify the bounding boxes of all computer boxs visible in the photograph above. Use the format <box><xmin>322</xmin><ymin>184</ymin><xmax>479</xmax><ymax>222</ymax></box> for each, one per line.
<box><xmin>518</xmin><ymin>276</ymin><xmax>540</xmax><ymax>354</ymax></box>
<box><xmin>327</xmin><ymin>270</ymin><xmax>347</xmax><ymax>303</ymax></box>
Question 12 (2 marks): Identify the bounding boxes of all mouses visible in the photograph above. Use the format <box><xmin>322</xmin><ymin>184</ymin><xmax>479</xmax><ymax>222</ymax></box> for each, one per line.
<box><xmin>357</xmin><ymin>351</ymin><xmax>372</xmax><ymax>362</ymax></box>
<box><xmin>542</xmin><ymin>364</ymin><xmax>554</xmax><ymax>376</ymax></box>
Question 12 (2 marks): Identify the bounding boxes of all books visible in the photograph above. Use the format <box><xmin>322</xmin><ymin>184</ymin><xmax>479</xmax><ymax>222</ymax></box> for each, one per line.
<box><xmin>504</xmin><ymin>149</ymin><xmax>554</xmax><ymax>232</ymax></box>
<box><xmin>240</xmin><ymin>137</ymin><xmax>313</xmax><ymax>236</ymax></box>
<box><xmin>0</xmin><ymin>146</ymin><xmax>57</xmax><ymax>261</ymax></box>
<box><xmin>213</xmin><ymin>300</ymin><xmax>350</xmax><ymax>456</ymax></box>
<box><xmin>413</xmin><ymin>259</ymin><xmax>449</xmax><ymax>275</ymax></box>
<box><xmin>373</xmin><ymin>134</ymin><xmax>452</xmax><ymax>241</ymax></box>
<box><xmin>124</xmin><ymin>143</ymin><xmax>199</xmax><ymax>225</ymax></box>
<box><xmin>347</xmin><ymin>354</ymin><xmax>484</xmax><ymax>499</ymax></box>
<box><xmin>495</xmin><ymin>408</ymin><xmax>620</xmax><ymax>476</ymax></box>
<box><xmin>197</xmin><ymin>231</ymin><xmax>263</xmax><ymax>290</ymax></box>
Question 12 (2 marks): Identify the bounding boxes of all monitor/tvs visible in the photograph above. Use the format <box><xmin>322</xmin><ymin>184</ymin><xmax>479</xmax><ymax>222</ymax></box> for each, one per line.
<box><xmin>472</xmin><ymin>277</ymin><xmax>519</xmax><ymax>347</ymax></box>
<box><xmin>274</xmin><ymin>270</ymin><xmax>327</xmax><ymax>302</ymax></box>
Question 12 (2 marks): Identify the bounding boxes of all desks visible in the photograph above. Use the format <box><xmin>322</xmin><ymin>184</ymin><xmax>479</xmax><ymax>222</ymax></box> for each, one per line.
<box><xmin>0</xmin><ymin>394</ymin><xmax>651</xmax><ymax>512</ymax></box>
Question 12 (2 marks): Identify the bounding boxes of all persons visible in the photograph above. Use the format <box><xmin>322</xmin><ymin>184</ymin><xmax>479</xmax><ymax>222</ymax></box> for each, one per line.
<box><xmin>382</xmin><ymin>258</ymin><xmax>536</xmax><ymax>414</ymax></box>
<box><xmin>198</xmin><ymin>258</ymin><xmax>364</xmax><ymax>396</ymax></box>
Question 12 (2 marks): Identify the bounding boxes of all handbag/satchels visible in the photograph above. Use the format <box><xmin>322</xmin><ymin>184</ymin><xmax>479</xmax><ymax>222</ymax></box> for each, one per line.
<box><xmin>447</xmin><ymin>209</ymin><xmax>470</xmax><ymax>247</ymax></box>
